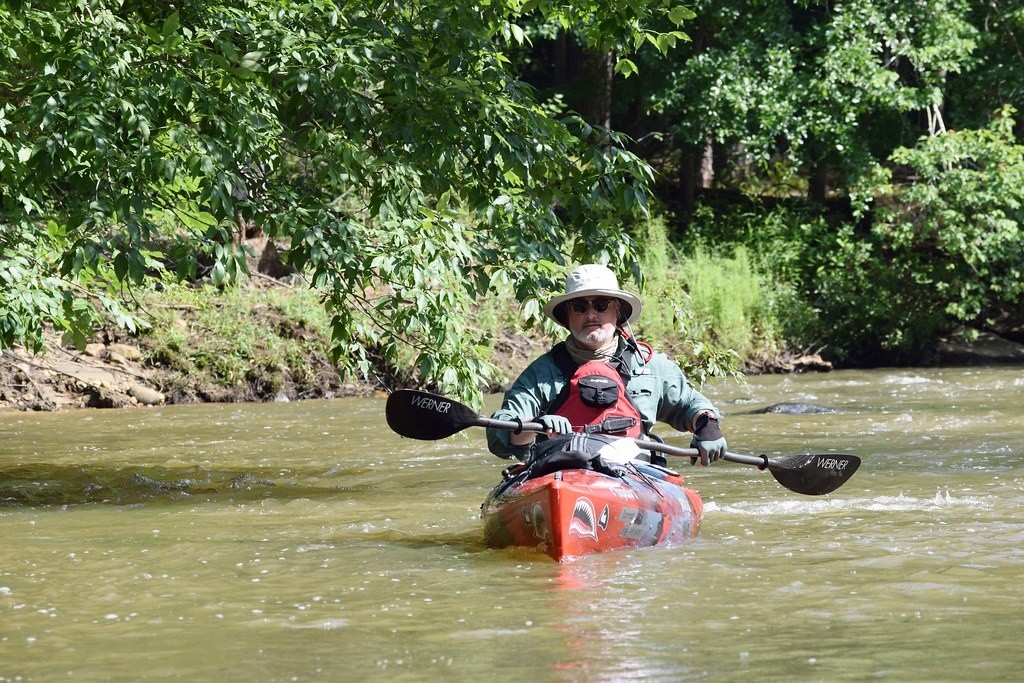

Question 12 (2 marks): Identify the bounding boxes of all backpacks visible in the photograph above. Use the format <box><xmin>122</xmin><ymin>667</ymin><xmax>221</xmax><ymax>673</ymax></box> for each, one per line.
<box><xmin>542</xmin><ymin>340</ymin><xmax>644</xmax><ymax>442</ymax></box>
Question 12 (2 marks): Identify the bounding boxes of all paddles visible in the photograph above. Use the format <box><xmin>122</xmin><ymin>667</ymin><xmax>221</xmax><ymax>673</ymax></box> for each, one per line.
<box><xmin>385</xmin><ymin>388</ymin><xmax>863</xmax><ymax>497</ymax></box>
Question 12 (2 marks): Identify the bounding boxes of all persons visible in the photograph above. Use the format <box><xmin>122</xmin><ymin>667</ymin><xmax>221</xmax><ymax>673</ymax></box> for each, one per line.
<box><xmin>486</xmin><ymin>263</ymin><xmax>727</xmax><ymax>486</ymax></box>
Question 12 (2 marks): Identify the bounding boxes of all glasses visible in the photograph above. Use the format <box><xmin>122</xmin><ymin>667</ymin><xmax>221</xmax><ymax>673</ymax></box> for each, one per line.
<box><xmin>569</xmin><ymin>297</ymin><xmax>614</xmax><ymax>313</ymax></box>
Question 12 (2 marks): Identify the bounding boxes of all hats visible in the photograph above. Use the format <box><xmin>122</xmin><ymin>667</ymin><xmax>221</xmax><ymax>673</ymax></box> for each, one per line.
<box><xmin>543</xmin><ymin>264</ymin><xmax>642</xmax><ymax>329</ymax></box>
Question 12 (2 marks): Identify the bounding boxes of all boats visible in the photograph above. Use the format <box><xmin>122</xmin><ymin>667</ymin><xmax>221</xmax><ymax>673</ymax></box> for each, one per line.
<box><xmin>480</xmin><ymin>460</ymin><xmax>705</xmax><ymax>565</ymax></box>
